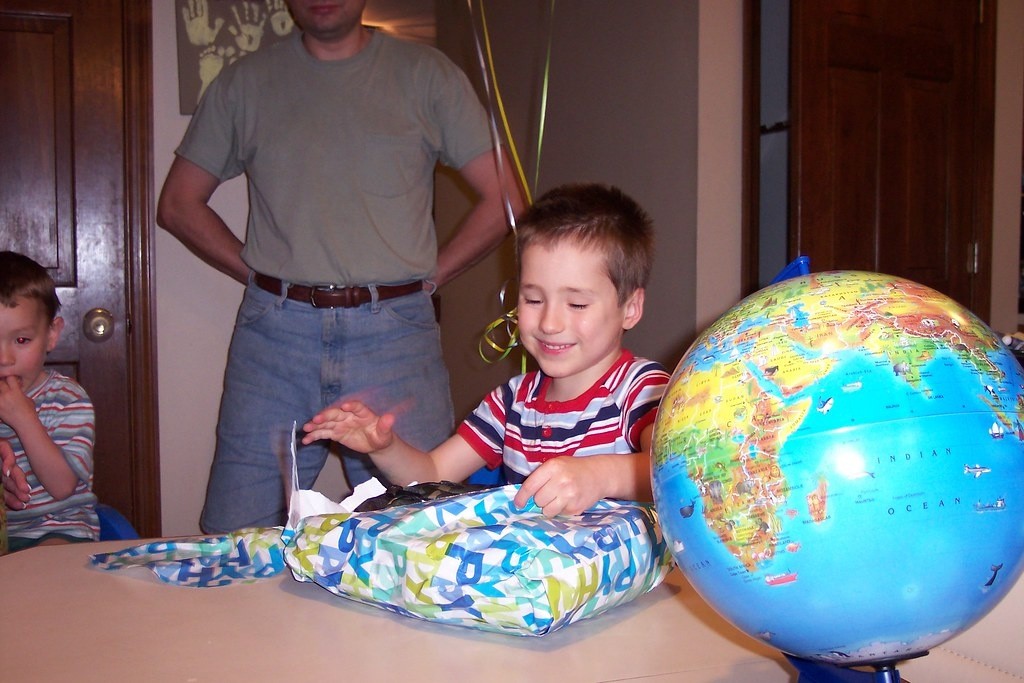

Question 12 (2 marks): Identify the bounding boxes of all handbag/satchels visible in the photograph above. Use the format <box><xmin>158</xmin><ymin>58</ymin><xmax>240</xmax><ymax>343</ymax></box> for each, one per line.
<box><xmin>283</xmin><ymin>484</ymin><xmax>676</xmax><ymax>638</ymax></box>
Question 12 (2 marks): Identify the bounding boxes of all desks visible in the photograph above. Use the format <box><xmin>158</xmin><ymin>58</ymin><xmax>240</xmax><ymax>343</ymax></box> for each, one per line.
<box><xmin>0</xmin><ymin>534</ymin><xmax>1023</xmax><ymax>682</ymax></box>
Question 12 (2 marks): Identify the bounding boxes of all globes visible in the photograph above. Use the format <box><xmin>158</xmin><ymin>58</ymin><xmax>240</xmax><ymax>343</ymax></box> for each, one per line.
<box><xmin>649</xmin><ymin>255</ymin><xmax>1024</xmax><ymax>683</ymax></box>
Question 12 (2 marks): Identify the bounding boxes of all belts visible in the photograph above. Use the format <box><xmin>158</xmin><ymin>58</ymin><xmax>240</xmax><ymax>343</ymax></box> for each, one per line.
<box><xmin>254</xmin><ymin>272</ymin><xmax>423</xmax><ymax>309</ymax></box>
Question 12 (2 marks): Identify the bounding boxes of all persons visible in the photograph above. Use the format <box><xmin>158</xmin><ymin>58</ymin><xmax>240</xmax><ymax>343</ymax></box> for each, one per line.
<box><xmin>301</xmin><ymin>181</ymin><xmax>671</xmax><ymax>518</ymax></box>
<box><xmin>0</xmin><ymin>251</ymin><xmax>98</xmax><ymax>556</ymax></box>
<box><xmin>155</xmin><ymin>0</ymin><xmax>528</xmax><ymax>536</ymax></box>
